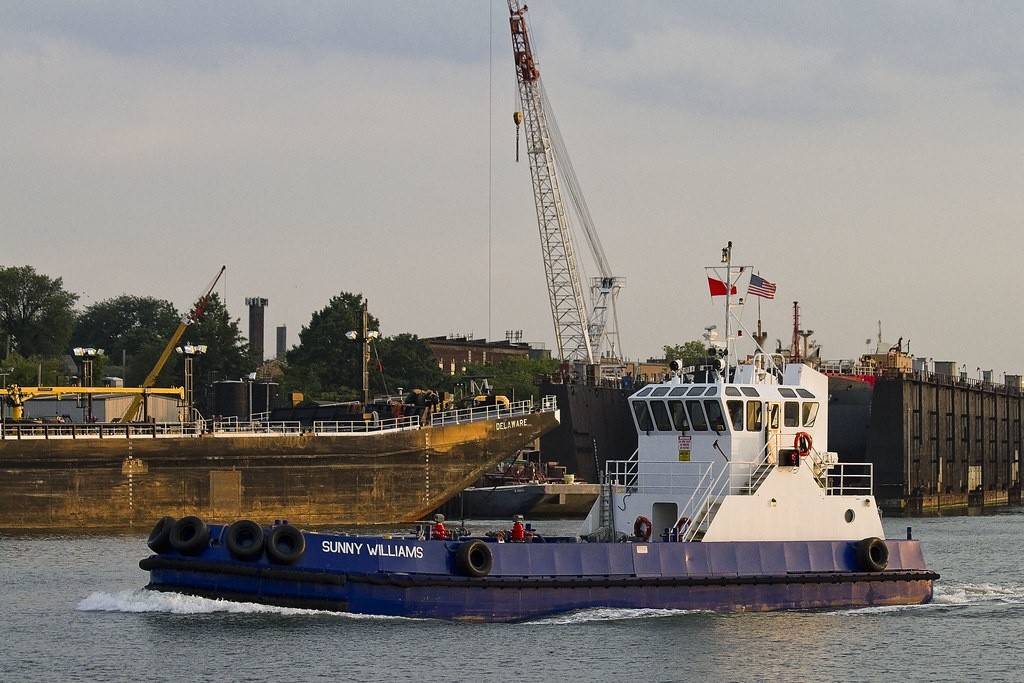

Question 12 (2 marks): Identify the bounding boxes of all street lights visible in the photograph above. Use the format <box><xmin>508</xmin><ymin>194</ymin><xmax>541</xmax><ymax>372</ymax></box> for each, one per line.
<box><xmin>73</xmin><ymin>348</ymin><xmax>104</xmax><ymax>434</ymax></box>
<box><xmin>175</xmin><ymin>340</ymin><xmax>208</xmax><ymax>422</ymax></box>
<box><xmin>247</xmin><ymin>370</ymin><xmax>256</xmax><ymax>426</ymax></box>
<box><xmin>345</xmin><ymin>298</ymin><xmax>379</xmax><ymax>403</ymax></box>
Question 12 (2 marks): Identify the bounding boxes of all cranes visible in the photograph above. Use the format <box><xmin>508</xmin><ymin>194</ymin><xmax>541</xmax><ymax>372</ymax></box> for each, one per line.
<box><xmin>506</xmin><ymin>0</ymin><xmax>627</xmax><ymax>383</ymax></box>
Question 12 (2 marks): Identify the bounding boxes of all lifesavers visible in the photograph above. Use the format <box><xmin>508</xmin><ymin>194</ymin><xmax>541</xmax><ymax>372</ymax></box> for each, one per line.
<box><xmin>634</xmin><ymin>516</ymin><xmax>651</xmax><ymax>540</ymax></box>
<box><xmin>459</xmin><ymin>540</ymin><xmax>494</xmax><ymax>576</ymax></box>
<box><xmin>854</xmin><ymin>537</ymin><xmax>890</xmax><ymax>572</ymax></box>
<box><xmin>145</xmin><ymin>515</ymin><xmax>211</xmax><ymax>558</ymax></box>
<box><xmin>675</xmin><ymin>518</ymin><xmax>691</xmax><ymax>531</ymax></box>
<box><xmin>794</xmin><ymin>432</ymin><xmax>812</xmax><ymax>456</ymax></box>
<box><xmin>226</xmin><ymin>520</ymin><xmax>304</xmax><ymax>566</ymax></box>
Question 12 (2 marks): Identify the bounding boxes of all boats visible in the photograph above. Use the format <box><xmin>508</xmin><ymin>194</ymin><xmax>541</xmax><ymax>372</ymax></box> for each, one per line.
<box><xmin>1</xmin><ymin>396</ymin><xmax>561</xmax><ymax>530</ymax></box>
<box><xmin>140</xmin><ymin>242</ymin><xmax>941</xmax><ymax>622</ymax></box>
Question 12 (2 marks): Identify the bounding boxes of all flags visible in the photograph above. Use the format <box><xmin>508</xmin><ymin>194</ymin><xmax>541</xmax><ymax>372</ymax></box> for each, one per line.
<box><xmin>748</xmin><ymin>275</ymin><xmax>776</xmax><ymax>298</ymax></box>
<box><xmin>707</xmin><ymin>277</ymin><xmax>738</xmax><ymax>295</ymax></box>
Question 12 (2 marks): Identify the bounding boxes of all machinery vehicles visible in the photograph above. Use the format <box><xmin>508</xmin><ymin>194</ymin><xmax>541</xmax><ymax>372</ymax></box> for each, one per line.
<box><xmin>2</xmin><ymin>386</ymin><xmax>185</xmax><ymax>433</ymax></box>
<box><xmin>111</xmin><ymin>266</ymin><xmax>228</xmax><ymax>431</ymax></box>
